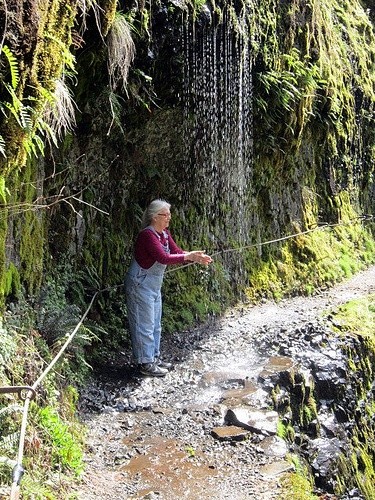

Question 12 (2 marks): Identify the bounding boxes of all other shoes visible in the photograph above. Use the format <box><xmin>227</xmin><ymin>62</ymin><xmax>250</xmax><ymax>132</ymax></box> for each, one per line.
<box><xmin>140</xmin><ymin>363</ymin><xmax>168</xmax><ymax>376</ymax></box>
<box><xmin>153</xmin><ymin>358</ymin><xmax>172</xmax><ymax>369</ymax></box>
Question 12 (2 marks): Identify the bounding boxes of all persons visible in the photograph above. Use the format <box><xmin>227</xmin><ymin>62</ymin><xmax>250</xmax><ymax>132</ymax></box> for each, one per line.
<box><xmin>123</xmin><ymin>200</ymin><xmax>214</xmax><ymax>378</ymax></box>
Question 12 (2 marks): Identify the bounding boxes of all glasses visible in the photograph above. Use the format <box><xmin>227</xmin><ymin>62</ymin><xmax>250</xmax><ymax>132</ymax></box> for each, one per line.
<box><xmin>157</xmin><ymin>213</ymin><xmax>172</xmax><ymax>217</ymax></box>
